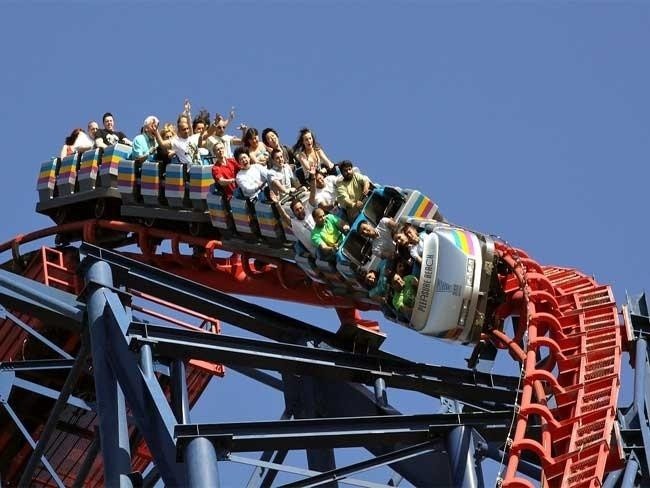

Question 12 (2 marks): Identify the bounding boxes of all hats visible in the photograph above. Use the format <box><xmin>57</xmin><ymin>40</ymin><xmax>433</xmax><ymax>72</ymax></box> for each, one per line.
<box><xmin>358</xmin><ymin>266</ymin><xmax>371</xmax><ymax>281</ymax></box>
<box><xmin>143</xmin><ymin>116</ymin><xmax>159</xmax><ymax>127</ymax></box>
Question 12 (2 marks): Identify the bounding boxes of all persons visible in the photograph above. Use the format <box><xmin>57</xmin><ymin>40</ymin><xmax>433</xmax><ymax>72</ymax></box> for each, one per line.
<box><xmin>133</xmin><ymin>98</ymin><xmax>267</xmax><ymax>235</ymax></box>
<box><xmin>261</xmin><ymin>126</ymin><xmax>426</xmax><ymax>320</ymax></box>
<box><xmin>61</xmin><ymin>113</ymin><xmax>132</xmax><ymax>187</ymax></box>
